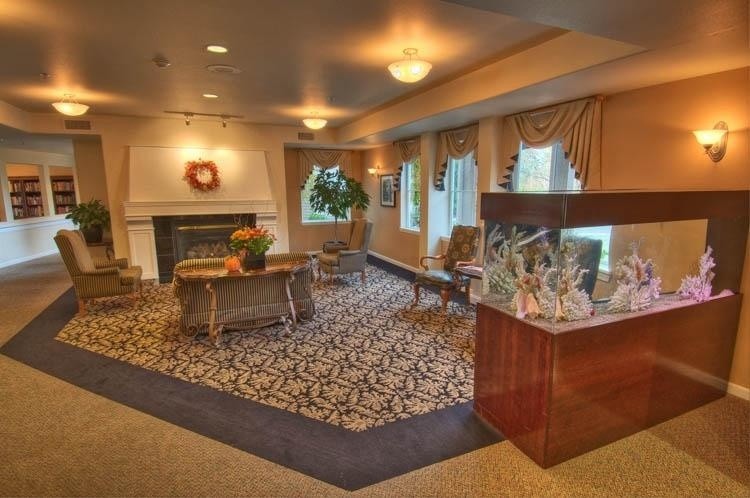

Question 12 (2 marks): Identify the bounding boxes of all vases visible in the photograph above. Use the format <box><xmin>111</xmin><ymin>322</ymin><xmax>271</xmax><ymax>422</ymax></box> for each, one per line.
<box><xmin>243</xmin><ymin>254</ymin><xmax>265</xmax><ymax>270</ymax></box>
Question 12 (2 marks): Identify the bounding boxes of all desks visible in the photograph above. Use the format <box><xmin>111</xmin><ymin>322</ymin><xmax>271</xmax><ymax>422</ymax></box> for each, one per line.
<box><xmin>177</xmin><ymin>260</ymin><xmax>310</xmax><ymax>347</ymax></box>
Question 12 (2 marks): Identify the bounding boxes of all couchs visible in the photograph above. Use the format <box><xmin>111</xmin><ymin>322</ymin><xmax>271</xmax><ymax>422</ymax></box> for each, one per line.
<box><xmin>172</xmin><ymin>251</ymin><xmax>315</xmax><ymax>337</ymax></box>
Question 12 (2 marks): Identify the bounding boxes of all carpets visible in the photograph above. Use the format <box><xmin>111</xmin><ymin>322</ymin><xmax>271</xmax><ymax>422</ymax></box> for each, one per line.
<box><xmin>2</xmin><ymin>275</ymin><xmax>509</xmax><ymax>493</ymax></box>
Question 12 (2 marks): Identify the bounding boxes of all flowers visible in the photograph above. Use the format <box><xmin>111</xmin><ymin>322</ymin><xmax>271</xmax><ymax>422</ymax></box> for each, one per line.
<box><xmin>229</xmin><ymin>226</ymin><xmax>273</xmax><ymax>255</ymax></box>
<box><xmin>183</xmin><ymin>160</ymin><xmax>221</xmax><ymax>192</ymax></box>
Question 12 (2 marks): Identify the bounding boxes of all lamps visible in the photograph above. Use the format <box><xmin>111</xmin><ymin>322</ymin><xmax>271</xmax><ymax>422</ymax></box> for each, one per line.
<box><xmin>51</xmin><ymin>93</ymin><xmax>89</xmax><ymax>117</ymax></box>
<box><xmin>302</xmin><ymin>111</ymin><xmax>328</xmax><ymax>130</ymax></box>
<box><xmin>692</xmin><ymin>121</ymin><xmax>728</xmax><ymax>162</ymax></box>
<box><xmin>387</xmin><ymin>48</ymin><xmax>434</xmax><ymax>83</ymax></box>
<box><xmin>164</xmin><ymin>111</ymin><xmax>244</xmax><ymax>127</ymax></box>
<box><xmin>368</xmin><ymin>165</ymin><xmax>379</xmax><ymax>179</ymax></box>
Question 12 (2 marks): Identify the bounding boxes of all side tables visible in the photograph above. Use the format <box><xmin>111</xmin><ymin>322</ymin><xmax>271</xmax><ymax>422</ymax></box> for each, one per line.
<box><xmin>456</xmin><ymin>266</ymin><xmax>483</xmax><ymax>281</ymax></box>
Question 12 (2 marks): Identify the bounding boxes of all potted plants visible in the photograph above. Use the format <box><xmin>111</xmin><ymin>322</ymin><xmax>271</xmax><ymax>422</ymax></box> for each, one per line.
<box><xmin>309</xmin><ymin>166</ymin><xmax>370</xmax><ymax>253</ymax></box>
<box><xmin>65</xmin><ymin>199</ymin><xmax>109</xmax><ymax>242</ymax></box>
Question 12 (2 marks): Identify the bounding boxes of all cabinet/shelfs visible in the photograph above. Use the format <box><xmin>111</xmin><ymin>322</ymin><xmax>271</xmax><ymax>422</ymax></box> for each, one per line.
<box><xmin>6</xmin><ymin>175</ymin><xmax>78</xmax><ymax>220</ymax></box>
<box><xmin>473</xmin><ymin>190</ymin><xmax>749</xmax><ymax>470</ymax></box>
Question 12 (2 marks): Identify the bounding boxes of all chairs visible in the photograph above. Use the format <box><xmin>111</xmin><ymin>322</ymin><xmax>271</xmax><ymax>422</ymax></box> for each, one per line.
<box><xmin>316</xmin><ymin>218</ymin><xmax>373</xmax><ymax>286</ymax></box>
<box><xmin>53</xmin><ymin>230</ymin><xmax>144</xmax><ymax>317</ymax></box>
<box><xmin>413</xmin><ymin>225</ymin><xmax>482</xmax><ymax>316</ymax></box>
<box><xmin>512</xmin><ymin>235</ymin><xmax>602</xmax><ymax>327</ymax></box>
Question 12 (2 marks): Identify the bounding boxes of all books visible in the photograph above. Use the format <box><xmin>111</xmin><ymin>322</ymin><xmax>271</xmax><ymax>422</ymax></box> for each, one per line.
<box><xmin>12</xmin><ymin>208</ymin><xmax>24</xmax><ymax>218</ymax></box>
<box><xmin>26</xmin><ymin>196</ymin><xmax>42</xmax><ymax>207</ymax></box>
<box><xmin>55</xmin><ymin>195</ymin><xmax>74</xmax><ymax>203</ymax></box>
<box><xmin>23</xmin><ymin>182</ymin><xmax>41</xmax><ymax>192</ymax></box>
<box><xmin>52</xmin><ymin>182</ymin><xmax>74</xmax><ymax>191</ymax></box>
<box><xmin>56</xmin><ymin>206</ymin><xmax>74</xmax><ymax>213</ymax></box>
<box><xmin>10</xmin><ymin>196</ymin><xmax>22</xmax><ymax>206</ymax></box>
<box><xmin>9</xmin><ymin>182</ymin><xmax>21</xmax><ymax>192</ymax></box>
<box><xmin>27</xmin><ymin>206</ymin><xmax>43</xmax><ymax>216</ymax></box>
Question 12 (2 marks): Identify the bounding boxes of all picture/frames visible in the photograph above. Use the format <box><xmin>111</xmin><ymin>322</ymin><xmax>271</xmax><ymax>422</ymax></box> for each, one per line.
<box><xmin>380</xmin><ymin>174</ymin><xmax>396</xmax><ymax>207</ymax></box>
